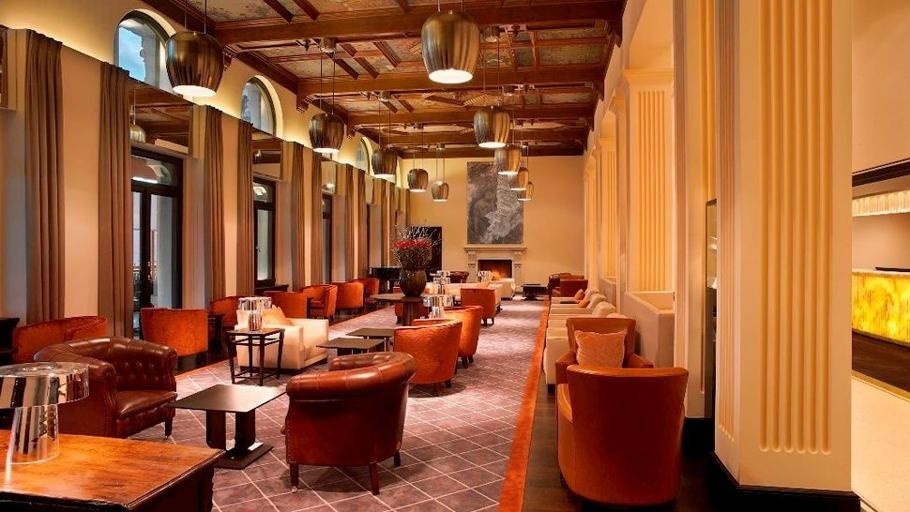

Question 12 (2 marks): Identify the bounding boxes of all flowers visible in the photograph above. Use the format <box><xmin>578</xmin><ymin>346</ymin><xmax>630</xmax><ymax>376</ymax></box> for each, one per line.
<box><xmin>388</xmin><ymin>217</ymin><xmax>441</xmax><ymax>268</ymax></box>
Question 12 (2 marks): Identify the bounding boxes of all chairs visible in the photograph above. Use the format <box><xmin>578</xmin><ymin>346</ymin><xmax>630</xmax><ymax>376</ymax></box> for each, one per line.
<box><xmin>32</xmin><ymin>336</ymin><xmax>178</xmax><ymax>444</ymax></box>
<box><xmin>12</xmin><ymin>314</ymin><xmax>108</xmax><ymax>365</ymax></box>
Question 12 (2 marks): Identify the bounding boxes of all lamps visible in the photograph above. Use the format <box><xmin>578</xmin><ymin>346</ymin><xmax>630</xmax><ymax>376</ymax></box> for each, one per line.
<box><xmin>164</xmin><ymin>0</ymin><xmax>223</xmax><ymax>98</ymax></box>
<box><xmin>421</xmin><ymin>1</ymin><xmax>482</xmax><ymax>85</ymax></box>
<box><xmin>305</xmin><ymin>41</ymin><xmax>535</xmax><ymax>204</ymax></box>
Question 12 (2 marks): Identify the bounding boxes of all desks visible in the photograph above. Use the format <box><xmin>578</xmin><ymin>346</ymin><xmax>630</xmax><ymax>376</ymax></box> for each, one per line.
<box><xmin>0</xmin><ymin>428</ymin><xmax>227</xmax><ymax>511</ymax></box>
<box><xmin>163</xmin><ymin>383</ymin><xmax>286</xmax><ymax>471</ymax></box>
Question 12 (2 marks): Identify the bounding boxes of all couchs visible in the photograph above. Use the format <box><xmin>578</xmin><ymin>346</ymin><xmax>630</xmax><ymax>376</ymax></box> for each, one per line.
<box><xmin>209</xmin><ymin>277</ymin><xmax>515</xmax><ymax>396</ymax></box>
<box><xmin>542</xmin><ymin>272</ymin><xmax>690</xmax><ymax>506</ymax></box>
<box><xmin>280</xmin><ymin>352</ymin><xmax>417</xmax><ymax>496</ymax></box>
<box><xmin>140</xmin><ymin>308</ymin><xmax>208</xmax><ymax>370</ymax></box>
